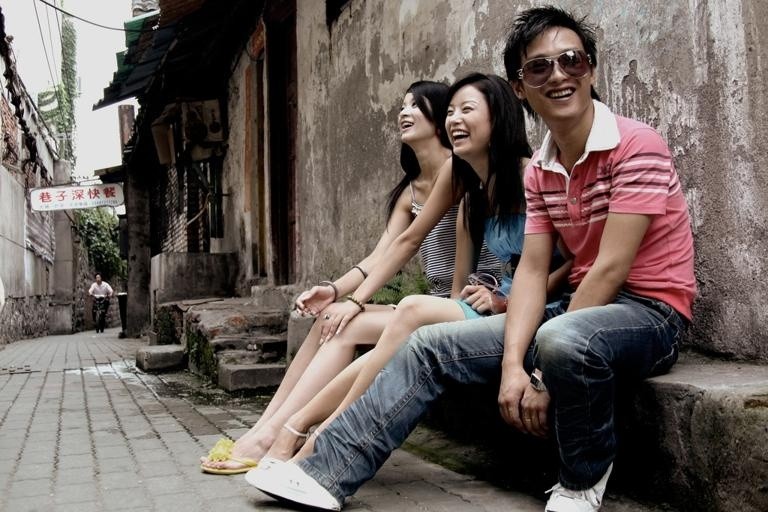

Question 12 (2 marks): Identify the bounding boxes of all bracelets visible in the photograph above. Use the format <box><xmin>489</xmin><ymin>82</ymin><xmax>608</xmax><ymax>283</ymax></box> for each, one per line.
<box><xmin>345</xmin><ymin>293</ymin><xmax>366</xmax><ymax>315</ymax></box>
<box><xmin>317</xmin><ymin>277</ymin><xmax>338</xmax><ymax>302</ymax></box>
<box><xmin>347</xmin><ymin>263</ymin><xmax>369</xmax><ymax>280</ymax></box>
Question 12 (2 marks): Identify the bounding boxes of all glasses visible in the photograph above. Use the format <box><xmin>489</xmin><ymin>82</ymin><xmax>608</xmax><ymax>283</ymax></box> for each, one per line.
<box><xmin>521</xmin><ymin>49</ymin><xmax>589</xmax><ymax>89</ymax></box>
<box><xmin>469</xmin><ymin>272</ymin><xmax>507</xmax><ymax>313</ymax></box>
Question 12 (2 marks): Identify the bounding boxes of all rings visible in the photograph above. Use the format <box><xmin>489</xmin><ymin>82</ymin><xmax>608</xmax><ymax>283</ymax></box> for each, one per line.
<box><xmin>523</xmin><ymin>417</ymin><xmax>532</xmax><ymax>423</ymax></box>
<box><xmin>322</xmin><ymin>314</ymin><xmax>330</xmax><ymax>321</ymax></box>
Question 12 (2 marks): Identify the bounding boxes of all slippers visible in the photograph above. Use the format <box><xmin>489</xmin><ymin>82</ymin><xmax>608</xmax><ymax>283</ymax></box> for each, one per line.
<box><xmin>200</xmin><ymin>439</ymin><xmax>257</xmax><ymax>475</ymax></box>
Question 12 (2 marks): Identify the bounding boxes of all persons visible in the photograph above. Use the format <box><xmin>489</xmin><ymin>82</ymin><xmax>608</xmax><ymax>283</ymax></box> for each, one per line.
<box><xmin>87</xmin><ymin>271</ymin><xmax>114</xmax><ymax>334</ymax></box>
<box><xmin>199</xmin><ymin>77</ymin><xmax>480</xmax><ymax>476</ymax></box>
<box><xmin>250</xmin><ymin>0</ymin><xmax>704</xmax><ymax>510</ymax></box>
<box><xmin>242</xmin><ymin>73</ymin><xmax>574</xmax><ymax>491</ymax></box>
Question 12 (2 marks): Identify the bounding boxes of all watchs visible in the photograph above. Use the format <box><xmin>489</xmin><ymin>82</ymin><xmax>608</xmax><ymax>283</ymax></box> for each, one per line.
<box><xmin>526</xmin><ymin>367</ymin><xmax>545</xmax><ymax>391</ymax></box>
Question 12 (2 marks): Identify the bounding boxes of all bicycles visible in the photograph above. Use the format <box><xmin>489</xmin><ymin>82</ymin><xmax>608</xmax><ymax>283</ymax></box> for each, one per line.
<box><xmin>87</xmin><ymin>294</ymin><xmax>112</xmax><ymax>334</ymax></box>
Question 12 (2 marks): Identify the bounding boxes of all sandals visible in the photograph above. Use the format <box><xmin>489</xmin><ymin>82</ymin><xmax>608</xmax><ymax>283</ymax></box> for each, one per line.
<box><xmin>245</xmin><ymin>419</ymin><xmax>311</xmax><ymax>483</ymax></box>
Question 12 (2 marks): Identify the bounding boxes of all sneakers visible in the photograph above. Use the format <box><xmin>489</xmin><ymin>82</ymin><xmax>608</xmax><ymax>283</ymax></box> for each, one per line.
<box><xmin>245</xmin><ymin>464</ymin><xmax>341</xmax><ymax>511</ymax></box>
<box><xmin>543</xmin><ymin>460</ymin><xmax>614</xmax><ymax>511</ymax></box>
